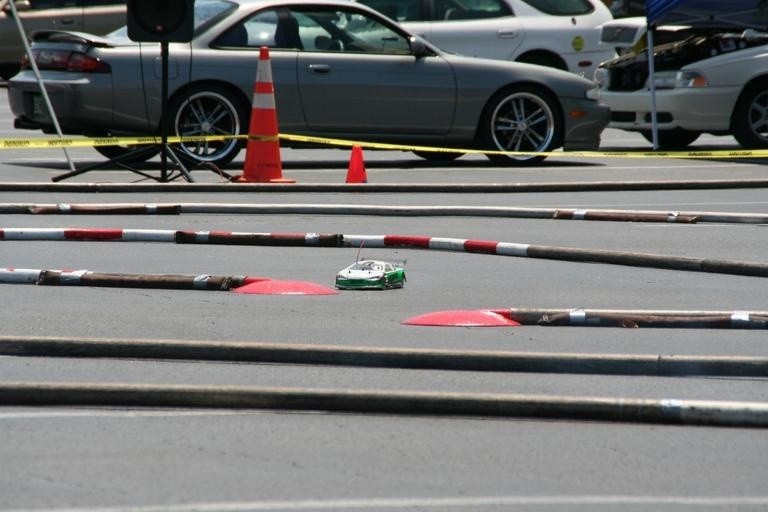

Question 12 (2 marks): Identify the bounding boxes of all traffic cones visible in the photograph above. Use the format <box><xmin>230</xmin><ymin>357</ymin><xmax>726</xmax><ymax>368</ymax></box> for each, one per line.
<box><xmin>233</xmin><ymin>47</ymin><xmax>295</xmax><ymax>185</ymax></box>
